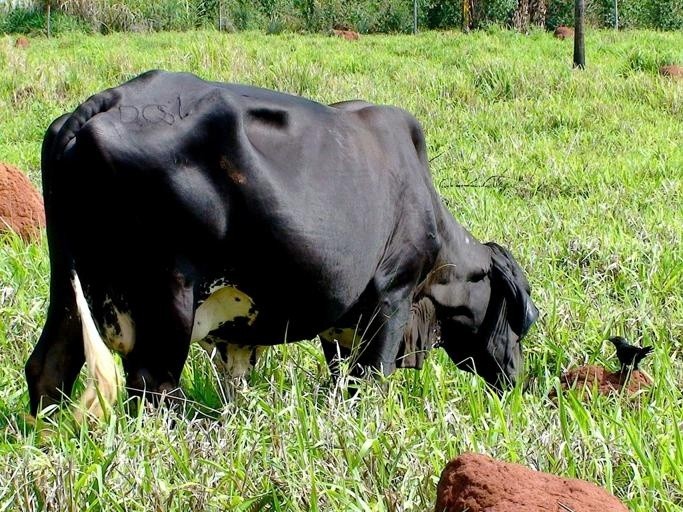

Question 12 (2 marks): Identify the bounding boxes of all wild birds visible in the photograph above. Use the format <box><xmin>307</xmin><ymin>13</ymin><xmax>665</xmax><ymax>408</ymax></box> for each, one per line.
<box><xmin>606</xmin><ymin>335</ymin><xmax>655</xmax><ymax>376</ymax></box>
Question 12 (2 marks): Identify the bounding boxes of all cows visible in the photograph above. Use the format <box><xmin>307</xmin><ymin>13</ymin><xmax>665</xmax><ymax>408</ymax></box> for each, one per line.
<box><xmin>24</xmin><ymin>69</ymin><xmax>540</xmax><ymax>444</ymax></box>
